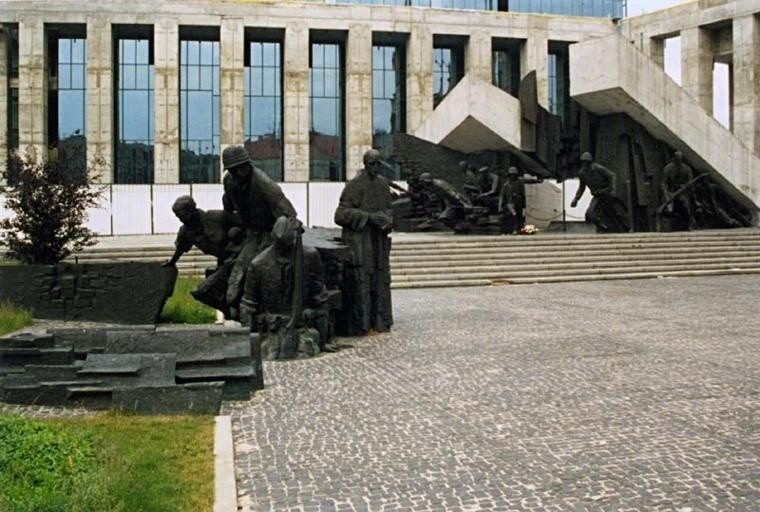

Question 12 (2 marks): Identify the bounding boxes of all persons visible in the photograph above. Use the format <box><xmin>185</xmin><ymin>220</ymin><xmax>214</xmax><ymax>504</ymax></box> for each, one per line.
<box><xmin>659</xmin><ymin>150</ymin><xmax>698</xmax><ymax>229</ymax></box>
<box><xmin>407</xmin><ymin>172</ymin><xmax>472</xmax><ymax>235</ymax></box>
<box><xmin>161</xmin><ymin>197</ymin><xmax>243</xmax><ymax>320</ymax></box>
<box><xmin>498</xmin><ymin>166</ymin><xmax>526</xmax><ymax>233</ymax></box>
<box><xmin>242</xmin><ymin>216</ymin><xmax>336</xmax><ymax>357</ymax></box>
<box><xmin>475</xmin><ymin>168</ymin><xmax>500</xmax><ymax>200</ymax></box>
<box><xmin>570</xmin><ymin>151</ymin><xmax>618</xmax><ymax>233</ymax></box>
<box><xmin>336</xmin><ymin>150</ymin><xmax>394</xmax><ymax>333</ymax></box>
<box><xmin>218</xmin><ymin>144</ymin><xmax>298</xmax><ymax>320</ymax></box>
